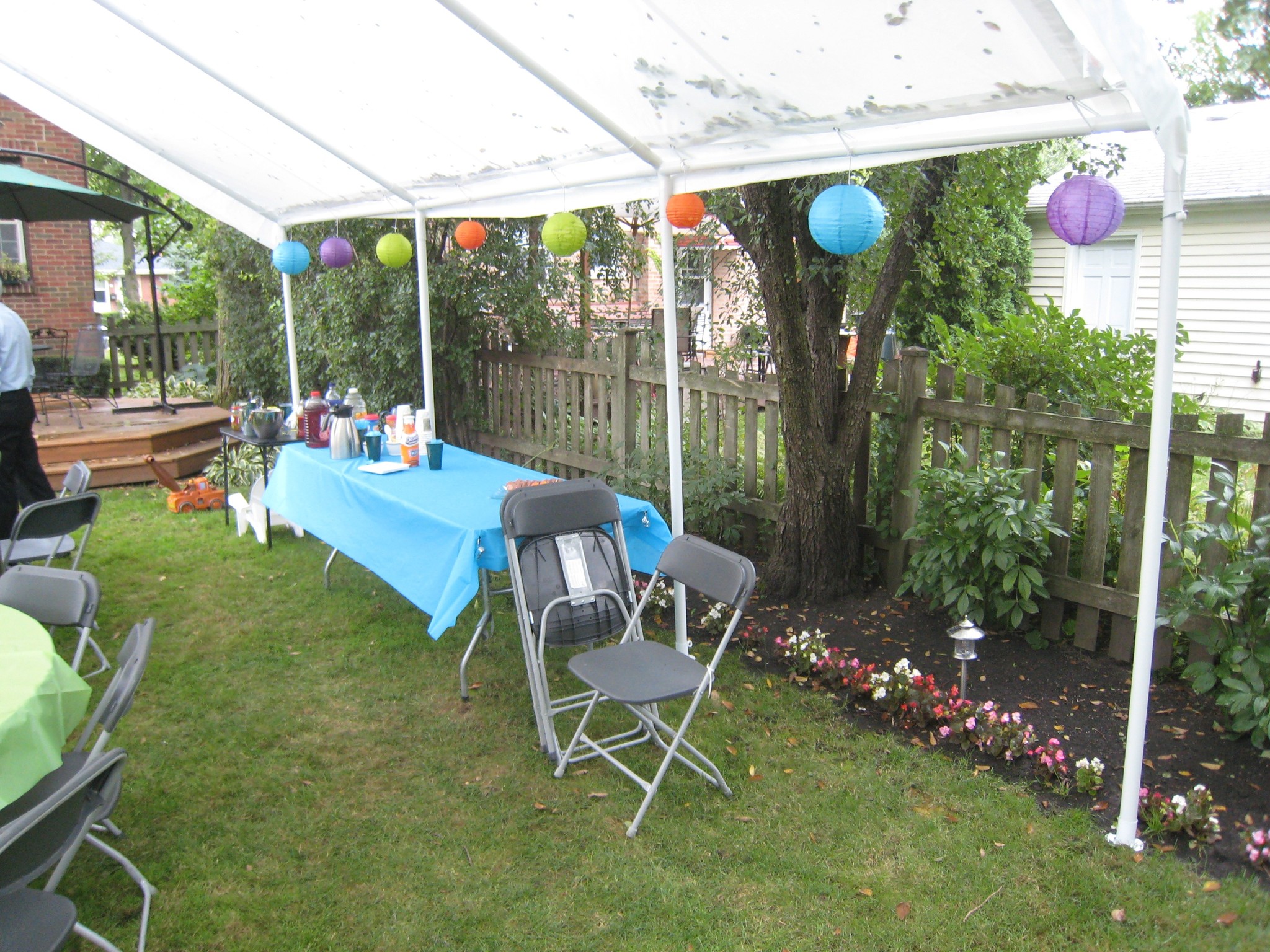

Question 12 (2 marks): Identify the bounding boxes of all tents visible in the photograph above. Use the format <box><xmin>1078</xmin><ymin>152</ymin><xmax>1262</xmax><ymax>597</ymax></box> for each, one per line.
<box><xmin>1</xmin><ymin>1</ymin><xmax>1202</xmax><ymax>856</ymax></box>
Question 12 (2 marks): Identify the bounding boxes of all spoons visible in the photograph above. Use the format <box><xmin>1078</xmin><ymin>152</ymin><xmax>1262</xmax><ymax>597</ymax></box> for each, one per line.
<box><xmin>383</xmin><ymin>424</ymin><xmax>392</xmax><ymax>441</ymax></box>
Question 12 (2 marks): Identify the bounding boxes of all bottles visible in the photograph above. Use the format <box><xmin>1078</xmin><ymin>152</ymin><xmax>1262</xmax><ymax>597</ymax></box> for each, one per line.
<box><xmin>396</xmin><ymin>404</ymin><xmax>411</xmax><ymax>441</ymax></box>
<box><xmin>229</xmin><ymin>404</ymin><xmax>305</xmax><ymax>438</ymax></box>
<box><xmin>365</xmin><ymin>414</ymin><xmax>380</xmax><ymax>432</ymax></box>
<box><xmin>385</xmin><ymin>414</ymin><xmax>397</xmax><ymax>441</ymax></box>
<box><xmin>400</xmin><ymin>415</ymin><xmax>420</xmax><ymax>467</ymax></box>
<box><xmin>303</xmin><ymin>382</ymin><xmax>367</xmax><ymax>449</ymax></box>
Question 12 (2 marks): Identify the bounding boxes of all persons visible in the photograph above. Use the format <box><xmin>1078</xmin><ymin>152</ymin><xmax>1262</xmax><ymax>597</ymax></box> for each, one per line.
<box><xmin>0</xmin><ymin>300</ymin><xmax>58</xmax><ymax>541</ymax></box>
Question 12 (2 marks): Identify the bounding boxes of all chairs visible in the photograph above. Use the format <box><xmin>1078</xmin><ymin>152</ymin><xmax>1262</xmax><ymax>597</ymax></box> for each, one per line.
<box><xmin>513</xmin><ymin>301</ymin><xmax>901</xmax><ymax>413</ymax></box>
<box><xmin>554</xmin><ymin>528</ymin><xmax>759</xmax><ymax>843</ymax></box>
<box><xmin>495</xmin><ymin>475</ymin><xmax>662</xmax><ymax>765</ymax></box>
<box><xmin>0</xmin><ymin>320</ymin><xmax>161</xmax><ymax>952</ymax></box>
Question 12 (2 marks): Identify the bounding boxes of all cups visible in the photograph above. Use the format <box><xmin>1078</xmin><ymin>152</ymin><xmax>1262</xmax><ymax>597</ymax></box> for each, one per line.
<box><xmin>414</xmin><ymin>409</ymin><xmax>444</xmax><ymax>471</ymax></box>
<box><xmin>364</xmin><ymin>431</ymin><xmax>381</xmax><ymax>461</ymax></box>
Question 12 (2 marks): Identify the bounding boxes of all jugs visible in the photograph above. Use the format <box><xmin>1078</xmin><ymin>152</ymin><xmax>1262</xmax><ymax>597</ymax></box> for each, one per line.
<box><xmin>321</xmin><ymin>404</ymin><xmax>362</xmax><ymax>459</ymax></box>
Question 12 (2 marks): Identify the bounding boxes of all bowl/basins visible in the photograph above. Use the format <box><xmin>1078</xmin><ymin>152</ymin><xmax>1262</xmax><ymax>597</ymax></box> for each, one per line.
<box><xmin>387</xmin><ymin>440</ymin><xmax>400</xmax><ymax>456</ymax></box>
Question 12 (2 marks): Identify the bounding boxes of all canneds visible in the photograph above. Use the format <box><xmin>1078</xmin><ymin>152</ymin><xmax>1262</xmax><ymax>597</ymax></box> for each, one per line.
<box><xmin>230</xmin><ymin>406</ymin><xmax>242</xmax><ymax>430</ymax></box>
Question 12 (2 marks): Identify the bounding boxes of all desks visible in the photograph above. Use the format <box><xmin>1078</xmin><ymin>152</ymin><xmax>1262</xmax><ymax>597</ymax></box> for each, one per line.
<box><xmin>215</xmin><ymin>424</ymin><xmax>313</xmax><ymax>551</ymax></box>
<box><xmin>257</xmin><ymin>424</ymin><xmax>680</xmax><ymax>709</ymax></box>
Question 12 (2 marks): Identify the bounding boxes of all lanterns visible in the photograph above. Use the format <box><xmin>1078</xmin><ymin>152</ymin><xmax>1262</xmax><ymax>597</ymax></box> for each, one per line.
<box><xmin>319</xmin><ymin>237</ymin><xmax>355</xmax><ymax>267</ymax></box>
<box><xmin>666</xmin><ymin>194</ymin><xmax>706</xmax><ymax>229</ymax></box>
<box><xmin>455</xmin><ymin>221</ymin><xmax>488</xmax><ymax>250</ymax></box>
<box><xmin>807</xmin><ymin>184</ymin><xmax>885</xmax><ymax>255</ymax></box>
<box><xmin>269</xmin><ymin>240</ymin><xmax>312</xmax><ymax>276</ymax></box>
<box><xmin>374</xmin><ymin>232</ymin><xmax>414</xmax><ymax>269</ymax></box>
<box><xmin>541</xmin><ymin>212</ymin><xmax>588</xmax><ymax>256</ymax></box>
<box><xmin>1045</xmin><ymin>173</ymin><xmax>1124</xmax><ymax>246</ymax></box>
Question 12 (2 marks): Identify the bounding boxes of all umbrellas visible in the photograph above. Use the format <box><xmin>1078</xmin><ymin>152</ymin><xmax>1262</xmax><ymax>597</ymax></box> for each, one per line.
<box><xmin>1</xmin><ymin>163</ymin><xmax>164</xmax><ymax>226</ymax></box>
